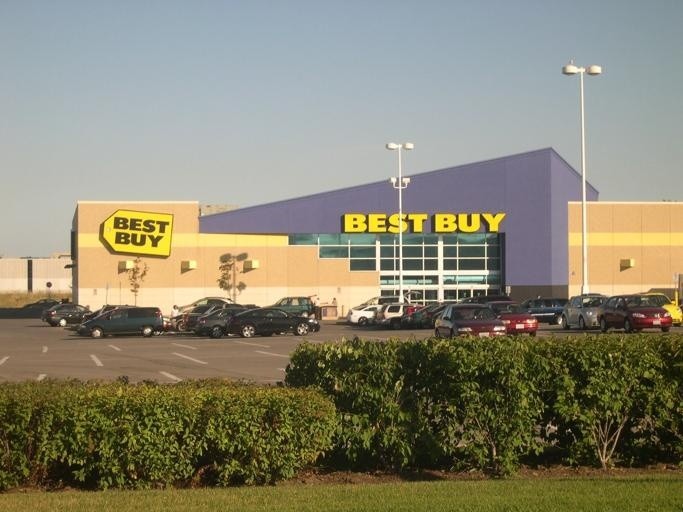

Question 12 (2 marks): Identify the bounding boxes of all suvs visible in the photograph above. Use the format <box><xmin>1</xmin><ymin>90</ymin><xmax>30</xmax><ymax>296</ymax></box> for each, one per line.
<box><xmin>363</xmin><ymin>295</ymin><xmax>411</xmax><ymax>306</ymax></box>
<box><xmin>513</xmin><ymin>297</ymin><xmax>565</xmax><ymax>326</ymax></box>
<box><xmin>377</xmin><ymin>303</ymin><xmax>421</xmax><ymax>329</ymax></box>
<box><xmin>459</xmin><ymin>295</ymin><xmax>511</xmax><ymax>303</ymax></box>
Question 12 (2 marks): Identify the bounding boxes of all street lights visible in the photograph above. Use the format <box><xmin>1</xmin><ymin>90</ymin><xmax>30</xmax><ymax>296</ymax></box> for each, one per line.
<box><xmin>564</xmin><ymin>62</ymin><xmax>603</xmax><ymax>294</ymax></box>
<box><xmin>385</xmin><ymin>140</ymin><xmax>415</xmax><ymax>303</ymax></box>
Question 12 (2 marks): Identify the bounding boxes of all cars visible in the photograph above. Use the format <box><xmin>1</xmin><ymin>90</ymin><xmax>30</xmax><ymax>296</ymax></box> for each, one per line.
<box><xmin>597</xmin><ymin>295</ymin><xmax>673</xmax><ymax>334</ymax></box>
<box><xmin>561</xmin><ymin>294</ymin><xmax>608</xmax><ymax>330</ymax></box>
<box><xmin>486</xmin><ymin>300</ymin><xmax>538</xmax><ymax>339</ymax></box>
<box><xmin>349</xmin><ymin>304</ymin><xmax>383</xmax><ymax>327</ymax></box>
<box><xmin>434</xmin><ymin>303</ymin><xmax>507</xmax><ymax>339</ymax></box>
<box><xmin>637</xmin><ymin>292</ymin><xmax>681</xmax><ymax>326</ymax></box>
<box><xmin>23</xmin><ymin>298</ymin><xmax>322</xmax><ymax>337</ymax></box>
<box><xmin>400</xmin><ymin>303</ymin><xmax>449</xmax><ymax>328</ymax></box>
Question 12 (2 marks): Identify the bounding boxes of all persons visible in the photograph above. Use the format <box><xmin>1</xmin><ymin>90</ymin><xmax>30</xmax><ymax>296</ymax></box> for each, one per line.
<box><xmin>313</xmin><ymin>298</ymin><xmax>320</xmax><ymax>322</ymax></box>
<box><xmin>170</xmin><ymin>304</ymin><xmax>177</xmax><ymax>330</ymax></box>
<box><xmin>330</xmin><ymin>298</ymin><xmax>336</xmax><ymax>306</ymax></box>
